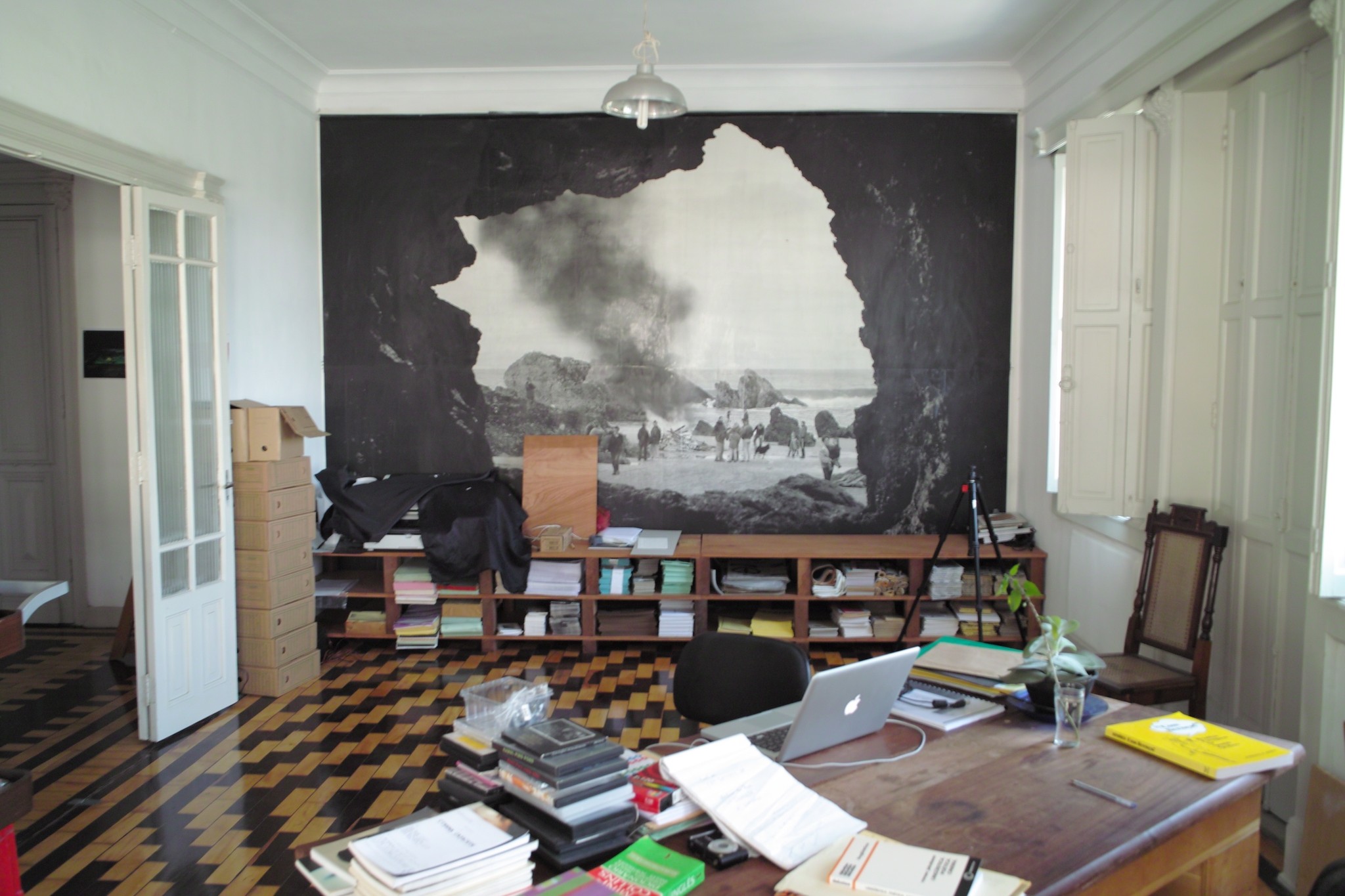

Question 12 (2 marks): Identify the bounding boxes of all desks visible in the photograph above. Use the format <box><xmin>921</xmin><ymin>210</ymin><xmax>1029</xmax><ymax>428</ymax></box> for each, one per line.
<box><xmin>293</xmin><ymin>693</ymin><xmax>1307</xmax><ymax>896</ymax></box>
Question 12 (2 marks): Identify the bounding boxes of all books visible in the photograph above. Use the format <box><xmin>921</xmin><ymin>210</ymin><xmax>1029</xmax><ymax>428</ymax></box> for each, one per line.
<box><xmin>1105</xmin><ymin>711</ymin><xmax>1295</xmax><ymax>780</ymax></box>
<box><xmin>392</xmin><ymin>556</ymin><xmax>1038</xmax><ymax>732</ymax></box>
<box><xmin>828</xmin><ymin>835</ymin><xmax>981</xmax><ymax>896</ymax></box>
<box><xmin>294</xmin><ymin>799</ymin><xmax>705</xmax><ymax>896</ymax></box>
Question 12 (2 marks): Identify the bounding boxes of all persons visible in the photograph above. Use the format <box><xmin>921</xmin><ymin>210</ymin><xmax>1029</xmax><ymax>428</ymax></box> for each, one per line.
<box><xmin>608</xmin><ymin>426</ymin><xmax>624</xmax><ymax>475</ymax></box>
<box><xmin>712</xmin><ymin>408</ymin><xmax>842</xmax><ymax>480</ymax></box>
<box><xmin>637</xmin><ymin>420</ymin><xmax>661</xmax><ymax>460</ymax></box>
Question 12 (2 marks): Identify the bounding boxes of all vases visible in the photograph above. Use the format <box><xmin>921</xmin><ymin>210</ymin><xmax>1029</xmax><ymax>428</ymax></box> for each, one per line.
<box><xmin>1053</xmin><ymin>688</ymin><xmax>1086</xmax><ymax>748</ymax></box>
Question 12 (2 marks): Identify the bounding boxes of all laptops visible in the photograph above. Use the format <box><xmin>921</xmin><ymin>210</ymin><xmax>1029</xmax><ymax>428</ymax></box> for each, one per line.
<box><xmin>700</xmin><ymin>645</ymin><xmax>919</xmax><ymax>764</ymax></box>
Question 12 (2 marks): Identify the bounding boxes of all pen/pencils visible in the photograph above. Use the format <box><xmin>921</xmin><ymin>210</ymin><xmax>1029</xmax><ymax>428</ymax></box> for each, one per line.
<box><xmin>1071</xmin><ymin>778</ymin><xmax>1138</xmax><ymax>809</ymax></box>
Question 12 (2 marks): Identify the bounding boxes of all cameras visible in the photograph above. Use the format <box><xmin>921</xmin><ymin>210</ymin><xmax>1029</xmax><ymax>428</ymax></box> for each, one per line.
<box><xmin>687</xmin><ymin>829</ymin><xmax>749</xmax><ymax>871</ymax></box>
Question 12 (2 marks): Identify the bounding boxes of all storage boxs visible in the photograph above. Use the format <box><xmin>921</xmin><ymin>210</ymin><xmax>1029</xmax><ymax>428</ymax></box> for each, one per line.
<box><xmin>230</xmin><ymin>398</ymin><xmax>331</xmax><ymax>698</ymax></box>
<box><xmin>540</xmin><ymin>525</ymin><xmax>572</xmax><ymax>552</ymax></box>
<box><xmin>463</xmin><ymin>675</ymin><xmax>554</xmax><ymax>732</ymax></box>
<box><xmin>344</xmin><ymin>602</ymin><xmax>387</xmax><ymax>636</ymax></box>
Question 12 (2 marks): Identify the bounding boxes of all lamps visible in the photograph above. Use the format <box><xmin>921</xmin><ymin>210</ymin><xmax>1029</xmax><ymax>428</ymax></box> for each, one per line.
<box><xmin>600</xmin><ymin>0</ymin><xmax>687</xmax><ymax>131</ymax></box>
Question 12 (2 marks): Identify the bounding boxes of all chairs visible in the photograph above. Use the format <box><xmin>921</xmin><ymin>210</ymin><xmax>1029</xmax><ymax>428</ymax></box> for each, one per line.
<box><xmin>672</xmin><ymin>634</ymin><xmax>811</xmax><ymax>724</ymax></box>
<box><xmin>1091</xmin><ymin>499</ymin><xmax>1229</xmax><ymax>721</ymax></box>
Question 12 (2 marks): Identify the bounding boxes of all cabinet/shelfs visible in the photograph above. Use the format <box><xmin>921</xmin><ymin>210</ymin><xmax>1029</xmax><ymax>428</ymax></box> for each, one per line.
<box><xmin>701</xmin><ymin>535</ymin><xmax>1048</xmax><ymax>648</ymax></box>
<box><xmin>313</xmin><ymin>533</ymin><xmax>701</xmax><ymax>655</ymax></box>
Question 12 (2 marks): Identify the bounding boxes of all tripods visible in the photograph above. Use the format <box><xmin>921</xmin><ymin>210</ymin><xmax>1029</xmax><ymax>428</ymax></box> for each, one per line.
<box><xmin>889</xmin><ymin>467</ymin><xmax>1027</xmax><ymax>655</ymax></box>
